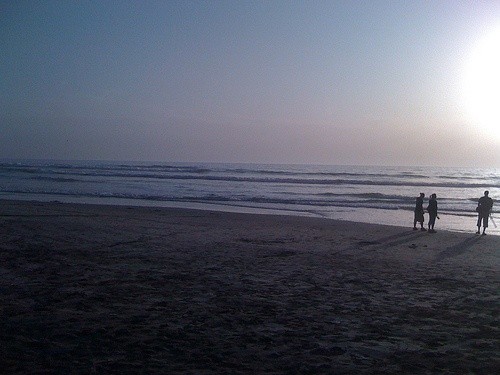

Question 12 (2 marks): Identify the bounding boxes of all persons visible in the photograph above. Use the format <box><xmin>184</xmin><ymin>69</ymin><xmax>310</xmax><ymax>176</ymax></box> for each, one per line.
<box><xmin>413</xmin><ymin>193</ymin><xmax>426</xmax><ymax>231</ymax></box>
<box><xmin>427</xmin><ymin>193</ymin><xmax>440</xmax><ymax>233</ymax></box>
<box><xmin>475</xmin><ymin>191</ymin><xmax>493</xmax><ymax>235</ymax></box>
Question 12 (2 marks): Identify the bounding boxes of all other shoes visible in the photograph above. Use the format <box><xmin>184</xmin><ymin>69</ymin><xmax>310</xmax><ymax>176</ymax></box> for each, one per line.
<box><xmin>428</xmin><ymin>229</ymin><xmax>433</xmax><ymax>232</ymax></box>
<box><xmin>475</xmin><ymin>231</ymin><xmax>480</xmax><ymax>234</ymax></box>
<box><xmin>412</xmin><ymin>227</ymin><xmax>418</xmax><ymax>231</ymax></box>
<box><xmin>432</xmin><ymin>229</ymin><xmax>437</xmax><ymax>232</ymax></box>
<box><xmin>482</xmin><ymin>232</ymin><xmax>488</xmax><ymax>235</ymax></box>
<box><xmin>420</xmin><ymin>228</ymin><xmax>427</xmax><ymax>231</ymax></box>
